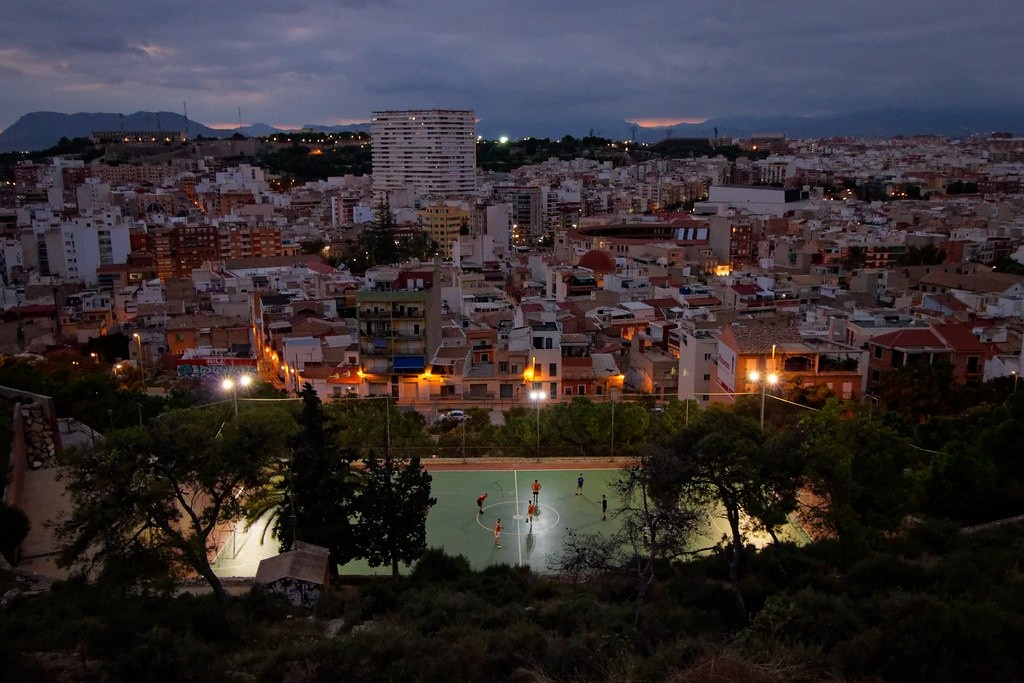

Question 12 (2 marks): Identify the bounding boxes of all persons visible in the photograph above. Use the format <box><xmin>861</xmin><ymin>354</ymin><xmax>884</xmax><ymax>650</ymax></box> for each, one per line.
<box><xmin>494</xmin><ymin>519</ymin><xmax>504</xmax><ymax>549</ymax></box>
<box><xmin>526</xmin><ymin>501</ymin><xmax>535</xmax><ymax>526</ymax></box>
<box><xmin>531</xmin><ymin>480</ymin><xmax>541</xmax><ymax>503</ymax></box>
<box><xmin>575</xmin><ymin>473</ymin><xmax>584</xmax><ymax>496</ymax></box>
<box><xmin>601</xmin><ymin>494</ymin><xmax>607</xmax><ymax>521</ymax></box>
<box><xmin>476</xmin><ymin>493</ymin><xmax>488</xmax><ymax>515</ymax></box>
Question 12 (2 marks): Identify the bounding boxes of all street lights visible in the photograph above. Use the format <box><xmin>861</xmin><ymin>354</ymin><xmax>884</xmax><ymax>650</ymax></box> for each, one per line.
<box><xmin>222</xmin><ymin>373</ymin><xmax>251</xmax><ymax>429</ymax></box>
<box><xmin>530</xmin><ymin>389</ymin><xmax>546</xmax><ymax>463</ymax></box>
<box><xmin>90</xmin><ymin>353</ymin><xmax>98</xmax><ymax>364</ymax></box>
<box><xmin>134</xmin><ymin>333</ymin><xmax>147</xmax><ymax>392</ymax></box>
<box><xmin>113</xmin><ymin>364</ymin><xmax>121</xmax><ymax>376</ymax></box>
<box><xmin>747</xmin><ymin>370</ymin><xmax>778</xmax><ymax>433</ymax></box>
<box><xmin>1012</xmin><ymin>370</ymin><xmax>1019</xmax><ymax>393</ymax></box>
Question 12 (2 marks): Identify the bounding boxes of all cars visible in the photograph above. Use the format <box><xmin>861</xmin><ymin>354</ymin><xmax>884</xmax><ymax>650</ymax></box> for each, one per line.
<box><xmin>438</xmin><ymin>410</ymin><xmax>472</xmax><ymax>421</ymax></box>
